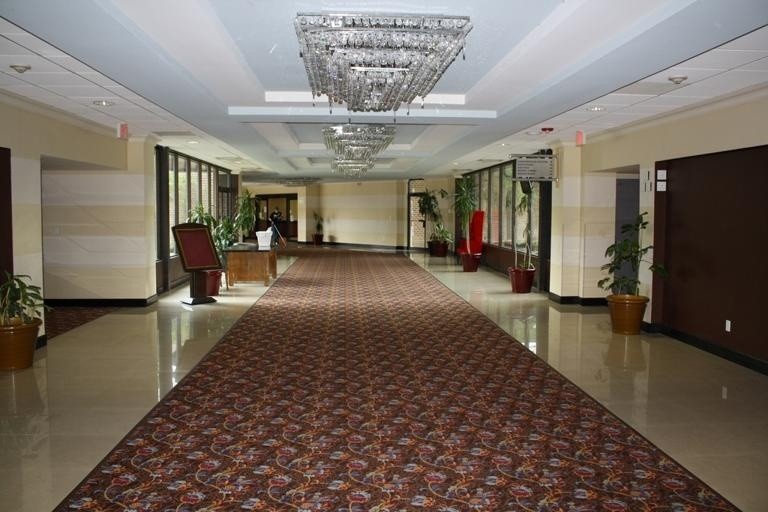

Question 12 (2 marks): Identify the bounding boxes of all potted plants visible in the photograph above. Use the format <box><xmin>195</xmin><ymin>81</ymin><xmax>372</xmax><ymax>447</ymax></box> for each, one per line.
<box><xmin>311</xmin><ymin>210</ymin><xmax>324</xmax><ymax>245</ymax></box>
<box><xmin>446</xmin><ymin>174</ymin><xmax>484</xmax><ymax>272</ymax></box>
<box><xmin>0</xmin><ymin>269</ymin><xmax>55</xmax><ymax>371</ymax></box>
<box><xmin>505</xmin><ymin>193</ymin><xmax>537</xmax><ymax>293</ymax></box>
<box><xmin>417</xmin><ymin>187</ymin><xmax>452</xmax><ymax>257</ymax></box>
<box><xmin>598</xmin><ymin>212</ymin><xmax>670</xmax><ymax>336</ymax></box>
<box><xmin>184</xmin><ymin>205</ymin><xmax>221</xmax><ymax>296</ymax></box>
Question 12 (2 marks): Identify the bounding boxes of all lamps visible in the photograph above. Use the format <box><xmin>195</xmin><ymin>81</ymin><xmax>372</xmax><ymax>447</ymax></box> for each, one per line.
<box><xmin>329</xmin><ymin>158</ymin><xmax>375</xmax><ymax>179</ymax></box>
<box><xmin>323</xmin><ymin>122</ymin><xmax>398</xmax><ymax>161</ymax></box>
<box><xmin>575</xmin><ymin>130</ymin><xmax>586</xmax><ymax>146</ymax></box>
<box><xmin>668</xmin><ymin>75</ymin><xmax>688</xmax><ymax>85</ymax></box>
<box><xmin>293</xmin><ymin>9</ymin><xmax>473</xmax><ymax>122</ymax></box>
<box><xmin>585</xmin><ymin>105</ymin><xmax>606</xmax><ymax>112</ymax></box>
<box><xmin>542</xmin><ymin>127</ymin><xmax>554</xmax><ymax>135</ymax></box>
<box><xmin>92</xmin><ymin>99</ymin><xmax>114</xmax><ymax>108</ymax></box>
<box><xmin>9</xmin><ymin>65</ymin><xmax>32</xmax><ymax>73</ymax></box>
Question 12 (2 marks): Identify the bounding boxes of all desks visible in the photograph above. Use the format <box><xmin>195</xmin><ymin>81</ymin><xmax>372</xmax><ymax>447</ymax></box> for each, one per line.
<box><xmin>220</xmin><ymin>243</ymin><xmax>277</xmax><ymax>286</ymax></box>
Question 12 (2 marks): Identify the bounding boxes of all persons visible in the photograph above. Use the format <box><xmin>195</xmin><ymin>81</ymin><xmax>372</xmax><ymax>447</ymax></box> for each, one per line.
<box><xmin>270</xmin><ymin>207</ymin><xmax>281</xmax><ymax>220</ymax></box>
